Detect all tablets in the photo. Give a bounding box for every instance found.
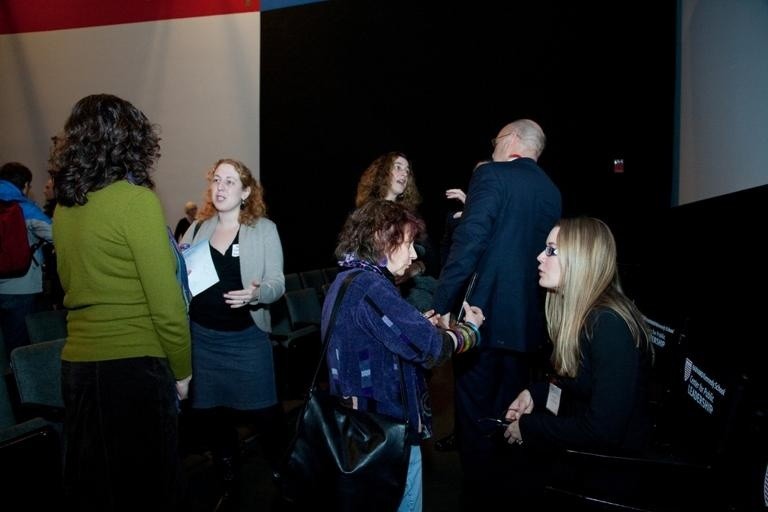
[445,272,479,326]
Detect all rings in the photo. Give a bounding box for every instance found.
[481,315,485,321]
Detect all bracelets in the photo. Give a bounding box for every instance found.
[448,321,481,354]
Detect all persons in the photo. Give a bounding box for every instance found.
[176,203,199,243]
[424,118,563,512]
[434,159,490,452]
[355,152,432,296]
[0,158,54,346]
[499,217,655,509]
[45,180,56,214]
[50,92,192,511]
[321,201,487,512]
[183,158,286,502]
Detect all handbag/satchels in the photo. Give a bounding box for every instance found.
[272,388,389,503]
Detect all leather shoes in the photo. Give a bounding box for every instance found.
[434,431,457,452]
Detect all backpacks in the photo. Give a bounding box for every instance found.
[0,199,33,280]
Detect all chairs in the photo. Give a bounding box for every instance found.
[543,309,736,512]
